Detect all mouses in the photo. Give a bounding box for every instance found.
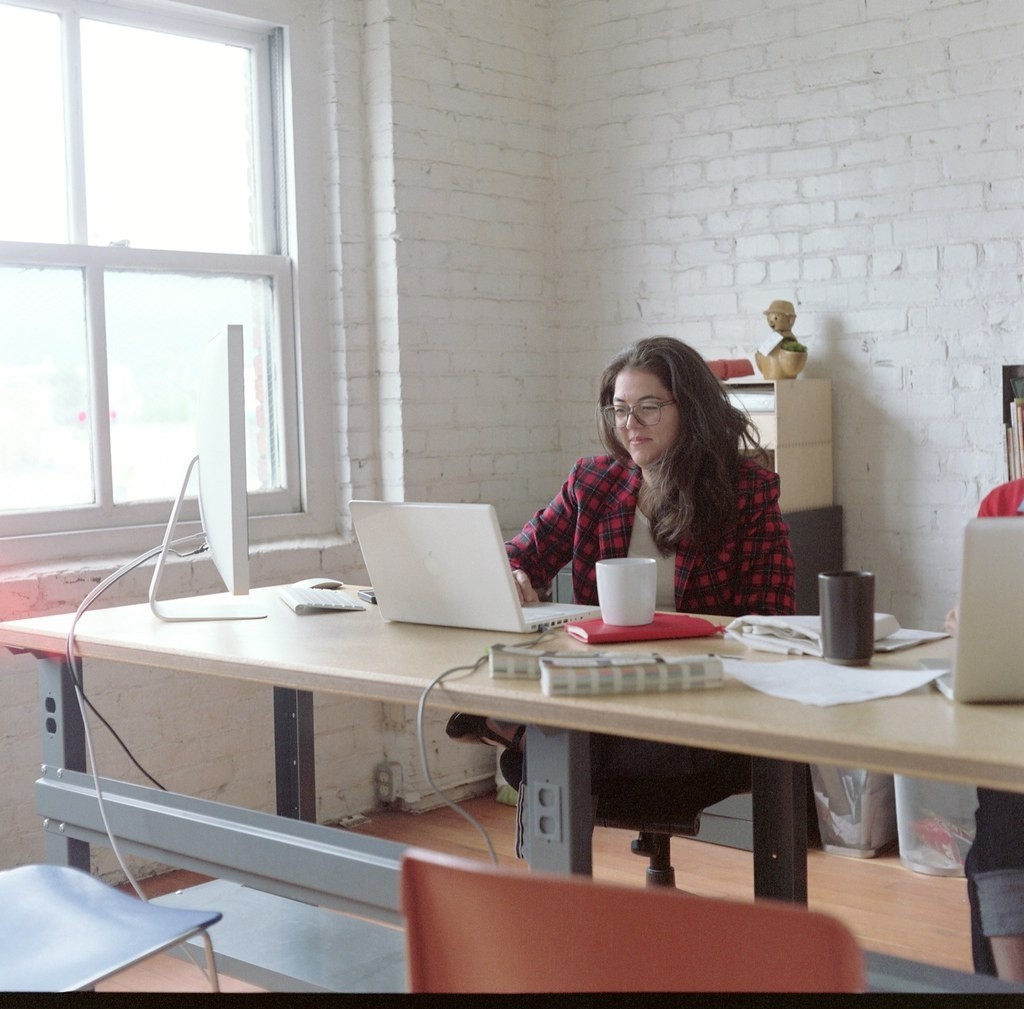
[295,578,344,590]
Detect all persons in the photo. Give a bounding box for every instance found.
[966,480,1024,984]
[446,336,796,795]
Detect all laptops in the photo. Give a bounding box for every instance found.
[349,500,602,634]
[922,516,1024,700]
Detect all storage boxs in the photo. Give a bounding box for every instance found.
[893,772,980,878]
[809,763,899,858]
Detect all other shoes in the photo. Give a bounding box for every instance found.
[445,711,527,753]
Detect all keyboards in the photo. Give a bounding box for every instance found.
[280,586,364,614]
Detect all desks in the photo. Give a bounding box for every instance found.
[0,583,1024,994]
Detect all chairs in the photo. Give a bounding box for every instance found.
[0,864,222,993]
[401,843,864,994]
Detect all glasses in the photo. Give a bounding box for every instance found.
[600,399,677,429]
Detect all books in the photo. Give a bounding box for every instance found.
[490,645,724,696]
[563,613,719,645]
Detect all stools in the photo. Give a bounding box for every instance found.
[496,748,752,891]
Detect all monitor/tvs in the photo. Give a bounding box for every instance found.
[148,324,270,622]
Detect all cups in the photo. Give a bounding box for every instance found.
[818,570,875,666]
[595,557,658,626]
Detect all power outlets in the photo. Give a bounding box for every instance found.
[376,762,402,802]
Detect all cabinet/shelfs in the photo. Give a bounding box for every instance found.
[674,379,833,849]
